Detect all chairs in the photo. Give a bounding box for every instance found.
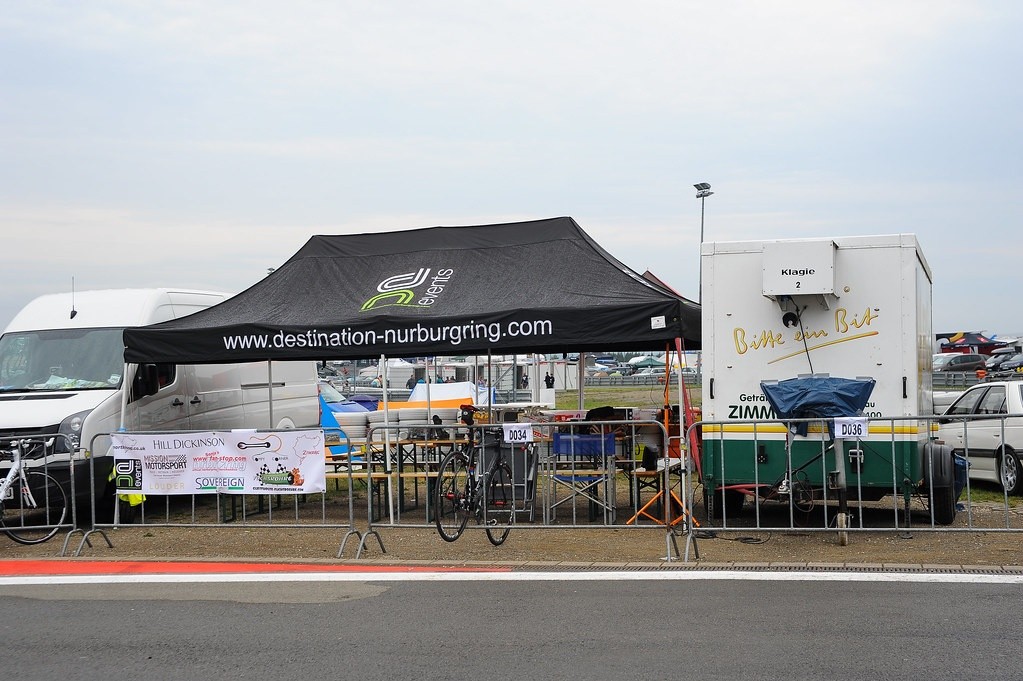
[547,432,617,524]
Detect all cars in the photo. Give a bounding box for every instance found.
[318,381,370,413]
[938,381,1023,493]
[985,354,1011,372]
[587,360,696,377]
[1000,353,1023,370]
[332,360,351,366]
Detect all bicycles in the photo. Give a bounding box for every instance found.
[1,437,67,545]
[434,404,516,546]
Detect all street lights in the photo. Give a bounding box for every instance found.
[693,182,714,305]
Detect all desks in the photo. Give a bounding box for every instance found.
[325,440,413,513]
[631,457,687,520]
[413,434,631,516]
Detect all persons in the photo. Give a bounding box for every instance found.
[461,376,467,382]
[478,376,484,386]
[343,367,348,374]
[544,372,555,388]
[521,373,528,389]
[437,374,456,383]
[370,375,382,388]
[424,376,431,383]
[405,375,424,389]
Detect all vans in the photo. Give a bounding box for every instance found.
[0,288,320,525]
[932,354,985,373]
[932,352,962,363]
[317,361,344,380]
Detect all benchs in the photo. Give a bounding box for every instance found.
[326,469,624,522]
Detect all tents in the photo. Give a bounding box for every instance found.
[114,217,701,524]
[940,334,1007,354]
[635,358,666,367]
